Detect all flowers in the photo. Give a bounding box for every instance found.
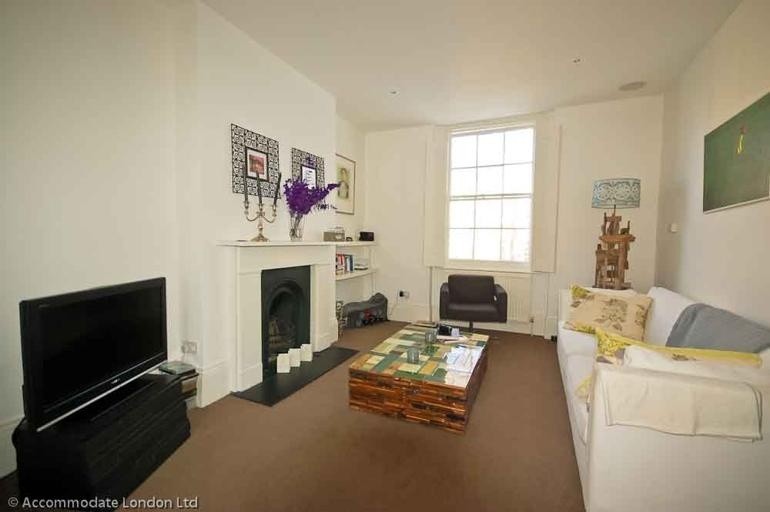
[283,176,340,236]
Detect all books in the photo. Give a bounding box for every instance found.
[336,253,354,275]
[353,265,368,270]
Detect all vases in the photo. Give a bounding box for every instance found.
[288,210,307,242]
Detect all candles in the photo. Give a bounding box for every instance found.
[256,170,262,204]
[241,164,248,202]
[273,172,281,205]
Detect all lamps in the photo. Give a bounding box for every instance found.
[592,178,640,290]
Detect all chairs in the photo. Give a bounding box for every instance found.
[439,274,508,328]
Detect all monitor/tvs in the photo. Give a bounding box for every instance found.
[19,276,169,434]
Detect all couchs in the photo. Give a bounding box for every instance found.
[556,285,770,512]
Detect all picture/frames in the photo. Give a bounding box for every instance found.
[335,153,356,215]
[300,164,317,190]
[244,146,269,182]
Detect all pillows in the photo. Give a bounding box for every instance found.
[623,345,770,386]
[574,326,763,398]
[562,281,652,343]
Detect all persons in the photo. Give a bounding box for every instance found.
[338,167,349,199]
[249,155,263,174]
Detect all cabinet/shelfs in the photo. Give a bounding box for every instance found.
[336,241,378,280]
[11,373,191,512]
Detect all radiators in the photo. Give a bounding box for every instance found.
[431,266,534,336]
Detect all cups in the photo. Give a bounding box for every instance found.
[408,348,419,363]
[425,330,437,345]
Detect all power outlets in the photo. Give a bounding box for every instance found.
[182,341,196,353]
